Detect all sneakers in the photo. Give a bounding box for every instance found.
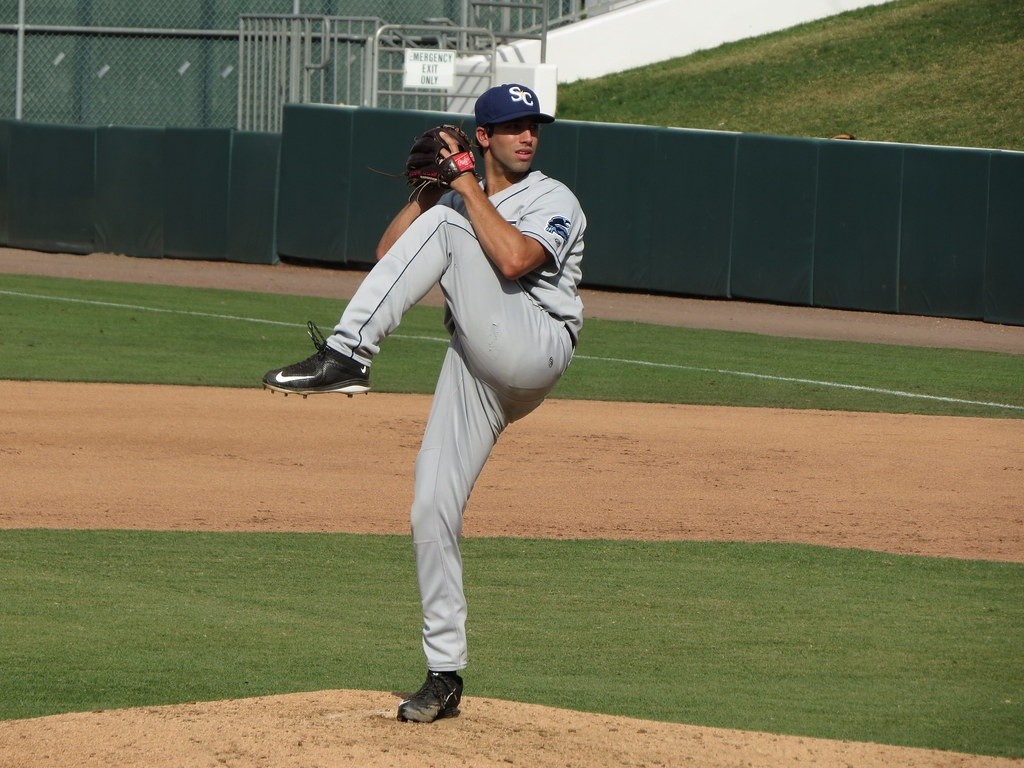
[260,320,372,400]
[397,671,462,723]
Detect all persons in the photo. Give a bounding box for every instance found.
[262,84,588,727]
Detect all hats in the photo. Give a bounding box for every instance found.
[474,83,554,128]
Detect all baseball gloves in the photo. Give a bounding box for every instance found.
[402,122,478,190]
